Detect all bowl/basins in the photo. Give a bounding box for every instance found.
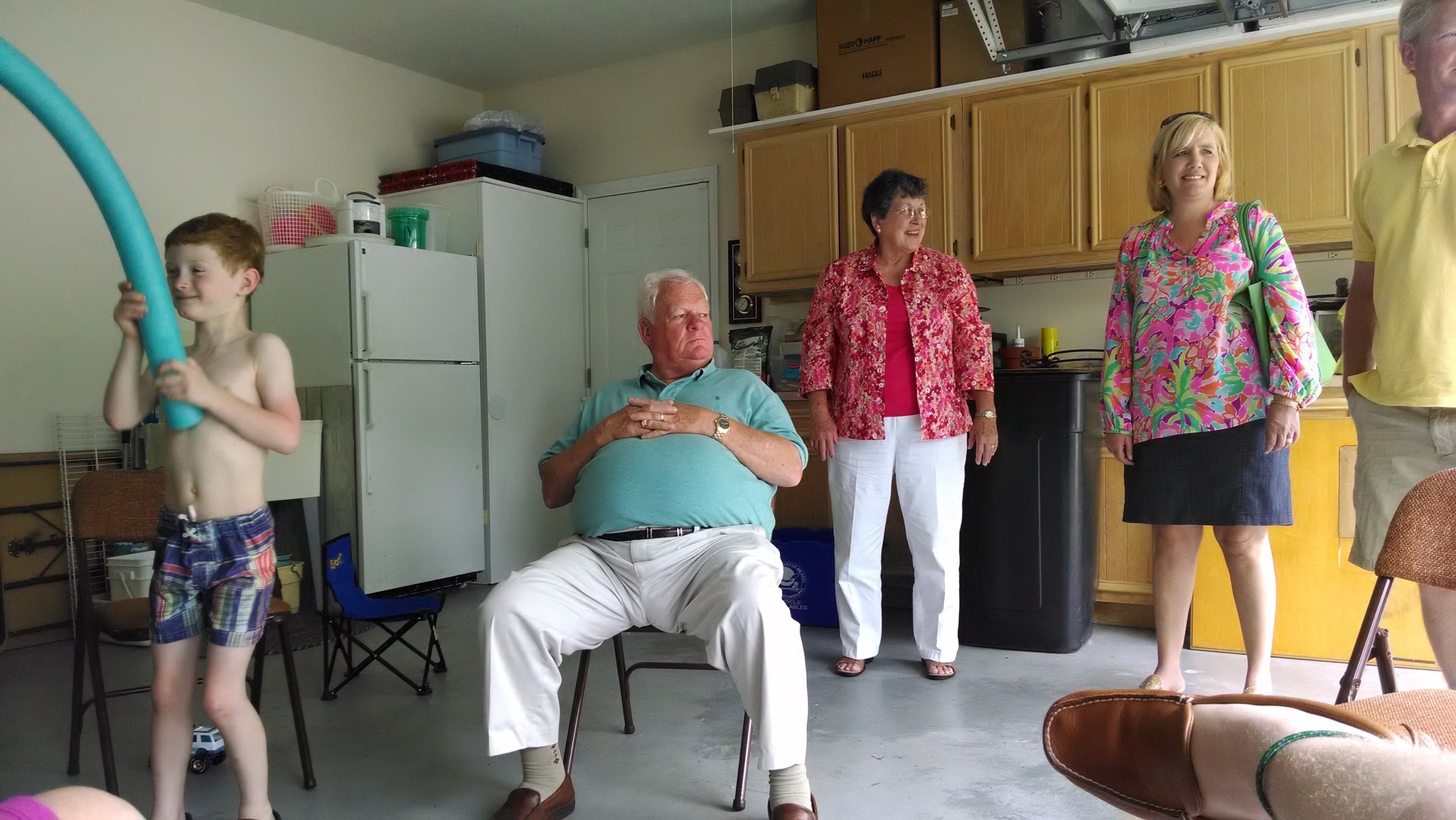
[998,347,1042,368]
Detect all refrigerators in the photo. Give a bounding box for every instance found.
[244,239,486,598]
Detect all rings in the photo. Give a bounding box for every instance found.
[991,445,995,448]
[1286,438,1293,444]
[660,413,663,421]
[642,420,647,428]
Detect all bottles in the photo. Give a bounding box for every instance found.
[713,341,733,369]
[1012,324,1024,349]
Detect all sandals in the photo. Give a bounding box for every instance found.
[835,657,874,677]
[921,657,956,679]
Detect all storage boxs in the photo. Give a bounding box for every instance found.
[754,59,818,120]
[718,84,759,127]
[940,0,1028,86]
[771,528,837,627]
[770,356,801,391]
[434,126,545,176]
[816,0,939,109]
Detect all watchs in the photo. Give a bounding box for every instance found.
[975,410,997,420]
[711,413,731,441]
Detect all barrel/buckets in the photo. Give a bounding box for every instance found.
[275,552,305,615]
[103,551,155,601]
[419,204,450,252]
[388,207,429,250]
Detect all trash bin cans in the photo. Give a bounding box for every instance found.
[959,369,1103,652]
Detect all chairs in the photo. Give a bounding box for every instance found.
[564,625,752,811]
[321,532,448,701]
[68,470,315,799]
[1336,468,1456,750]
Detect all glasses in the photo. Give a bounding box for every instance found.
[1161,112,1213,129]
[887,205,934,219]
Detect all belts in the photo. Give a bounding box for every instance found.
[599,526,705,542]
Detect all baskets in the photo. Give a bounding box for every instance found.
[258,178,341,253]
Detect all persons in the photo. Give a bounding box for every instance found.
[0,786,146,820]
[102,211,303,820]
[1096,110,1325,695]
[1043,690,1456,820]
[1339,0,1456,687]
[477,268,820,820]
[801,168,998,679]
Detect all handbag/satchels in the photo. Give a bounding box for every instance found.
[1235,203,1337,395]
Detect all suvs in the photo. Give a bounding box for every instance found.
[185,724,229,775]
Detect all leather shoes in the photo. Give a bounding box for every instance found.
[489,772,575,820]
[1042,690,1437,820]
[765,792,821,820]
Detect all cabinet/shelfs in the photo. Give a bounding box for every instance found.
[740,20,1421,297]
[379,176,585,584]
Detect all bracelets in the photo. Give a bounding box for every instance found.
[1270,400,1301,413]
